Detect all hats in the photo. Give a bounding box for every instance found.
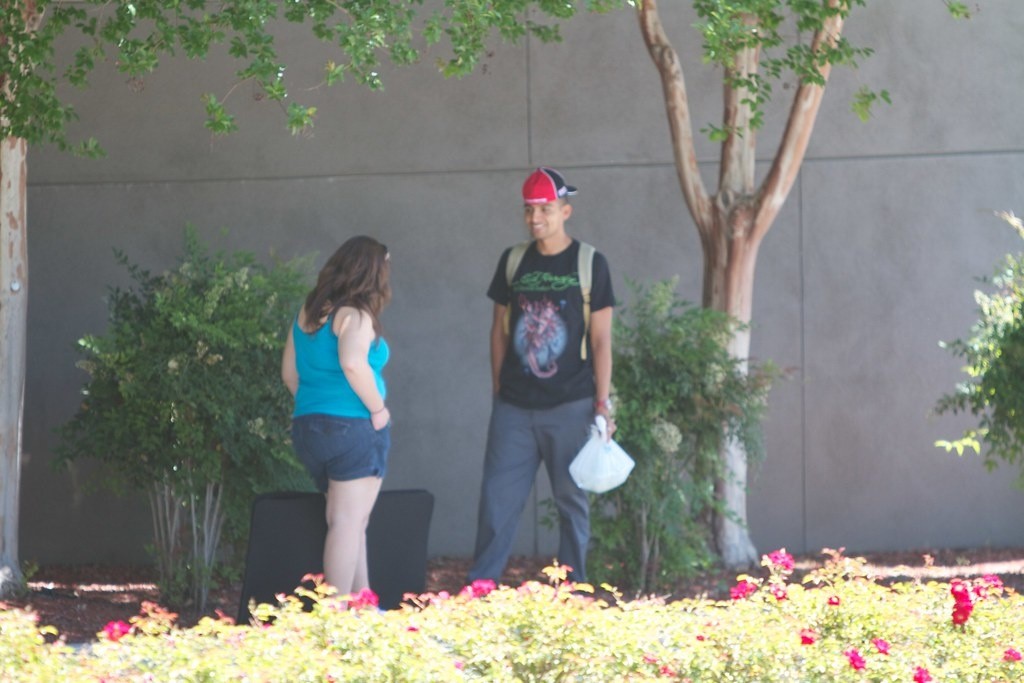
[522,166,578,205]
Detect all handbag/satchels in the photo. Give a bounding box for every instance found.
[569,401,635,494]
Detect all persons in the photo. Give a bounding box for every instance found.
[466,168,617,593]
[282,235,392,609]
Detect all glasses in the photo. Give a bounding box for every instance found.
[381,245,390,261]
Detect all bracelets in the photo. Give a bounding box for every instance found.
[370,406,386,415]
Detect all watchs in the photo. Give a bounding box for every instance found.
[593,399,612,411]
